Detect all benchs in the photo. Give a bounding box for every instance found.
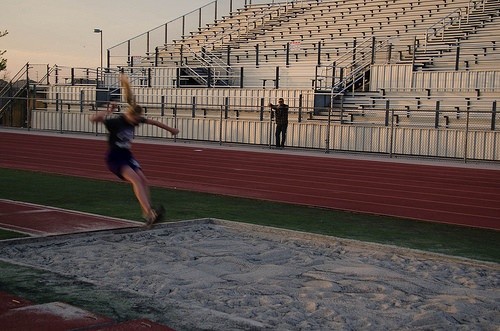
[36,0,500,131]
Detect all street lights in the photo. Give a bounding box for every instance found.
[93,28,103,87]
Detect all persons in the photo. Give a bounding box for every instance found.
[89,75,180,227]
[268,98,289,149]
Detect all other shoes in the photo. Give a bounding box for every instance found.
[146,207,166,227]
